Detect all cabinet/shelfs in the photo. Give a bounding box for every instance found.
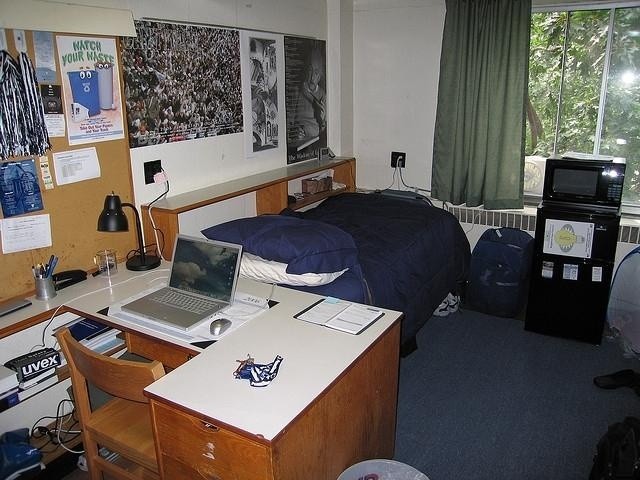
[141,157,356,262]
[524,202,623,344]
[0,262,404,480]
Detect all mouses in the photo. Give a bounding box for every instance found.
[210,318,232,336]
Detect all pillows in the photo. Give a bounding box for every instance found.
[199,213,356,289]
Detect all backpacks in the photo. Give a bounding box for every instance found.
[464,227,535,317]
[589,417,640,480]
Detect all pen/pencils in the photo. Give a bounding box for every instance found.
[32,254,58,278]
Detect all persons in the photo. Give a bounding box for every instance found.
[293,48,327,138]
[119,19,244,148]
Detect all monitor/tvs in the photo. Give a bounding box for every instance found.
[542,154,626,213]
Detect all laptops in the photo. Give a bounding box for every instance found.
[121,234,243,332]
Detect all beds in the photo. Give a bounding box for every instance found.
[251,191,467,322]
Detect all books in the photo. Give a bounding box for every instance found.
[68,317,128,359]
[18,367,59,402]
[292,296,385,335]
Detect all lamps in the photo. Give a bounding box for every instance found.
[96,193,160,272]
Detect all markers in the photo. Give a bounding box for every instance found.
[92,264,114,277]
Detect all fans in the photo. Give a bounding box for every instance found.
[524,156,546,195]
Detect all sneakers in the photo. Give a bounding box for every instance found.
[432,300,449,316]
[447,295,459,312]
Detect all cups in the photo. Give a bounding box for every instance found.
[95,248,117,276]
[35,276,57,301]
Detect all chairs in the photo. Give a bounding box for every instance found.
[55,329,166,480]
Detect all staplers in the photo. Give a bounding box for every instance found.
[53,270,87,291]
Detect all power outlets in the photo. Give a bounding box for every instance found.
[391,152,406,168]
[144,159,162,184]
